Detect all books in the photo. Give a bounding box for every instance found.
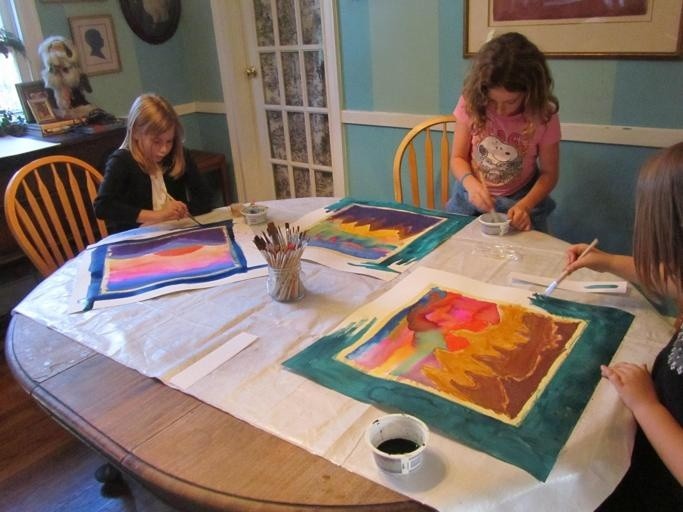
[70,118,127,134]
[23,118,82,138]
[52,103,98,120]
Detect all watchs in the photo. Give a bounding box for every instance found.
[457,173,474,192]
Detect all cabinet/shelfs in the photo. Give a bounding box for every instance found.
[0,131,129,269]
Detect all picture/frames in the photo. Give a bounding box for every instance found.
[15,81,44,123]
[26,88,55,109]
[120,0,182,46]
[27,99,56,124]
[463,0,683,62]
[68,14,122,77]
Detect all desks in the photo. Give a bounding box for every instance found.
[4,197,653,510]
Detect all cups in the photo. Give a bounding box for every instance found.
[267,263,305,302]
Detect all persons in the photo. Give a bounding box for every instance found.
[443,31,562,235]
[85,28,105,60]
[143,0,170,37]
[92,93,188,236]
[565,142,683,489]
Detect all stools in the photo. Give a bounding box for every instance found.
[189,148,232,207]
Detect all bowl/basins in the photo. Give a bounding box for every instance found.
[365,412,430,475]
[240,205,270,225]
[479,213,513,235]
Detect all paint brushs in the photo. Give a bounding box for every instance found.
[545,238,598,299]
[160,188,203,226]
[478,170,500,222]
[250,222,310,301]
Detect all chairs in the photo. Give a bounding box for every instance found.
[3,154,106,277]
[393,114,459,210]
